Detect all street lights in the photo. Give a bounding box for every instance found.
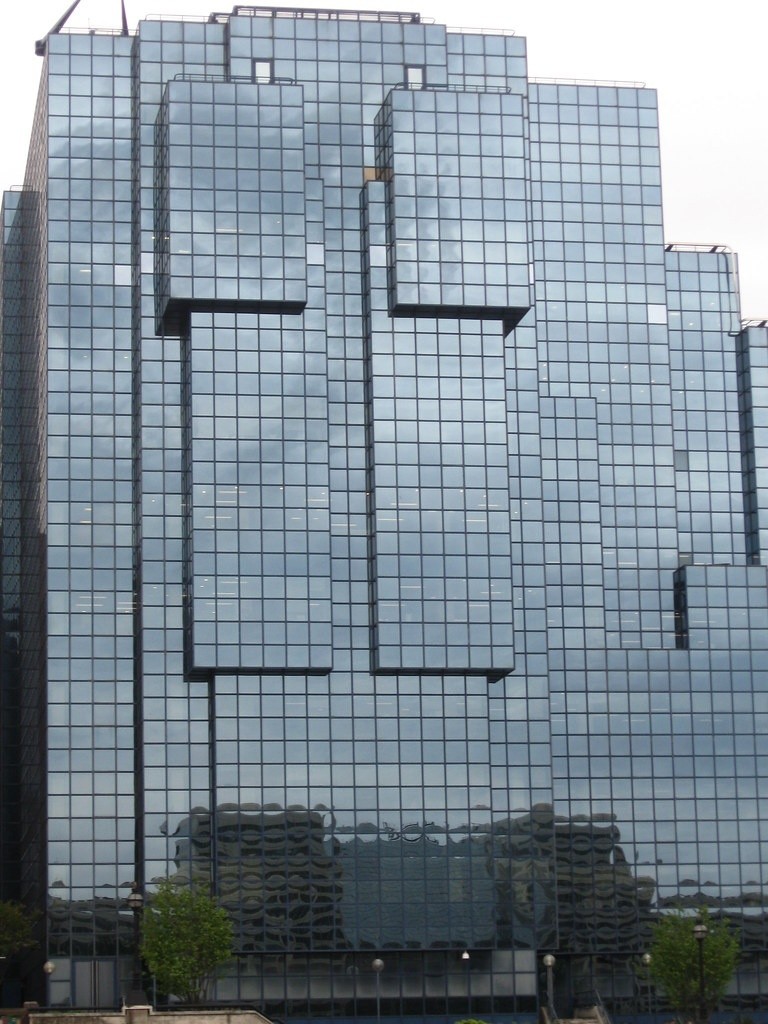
[691,916,710,1024]
[125,887,147,1006]
